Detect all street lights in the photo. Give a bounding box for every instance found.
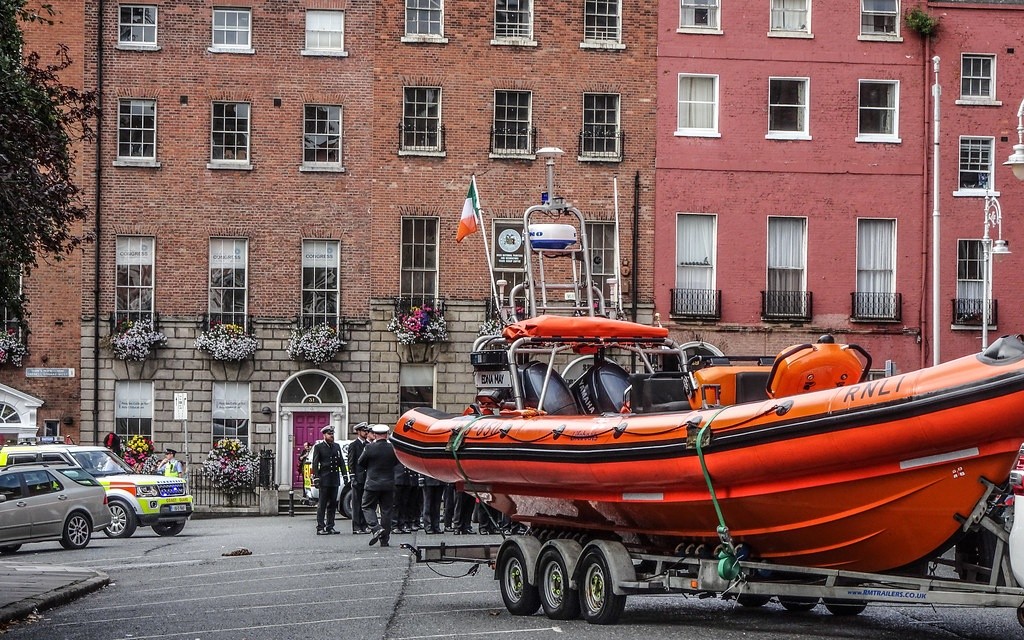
[979,166,1008,352]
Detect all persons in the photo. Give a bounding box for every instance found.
[298,441,310,498]
[348,421,528,535]
[312,426,348,535]
[358,424,395,547]
[157,449,182,478]
[104,432,120,457]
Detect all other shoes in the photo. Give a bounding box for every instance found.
[380,541,389,547]
[369,528,385,546]
[353,526,371,534]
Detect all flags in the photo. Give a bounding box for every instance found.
[456,180,479,243]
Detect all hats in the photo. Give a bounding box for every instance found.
[320,426,335,434]
[372,424,389,433]
[164,448,176,454]
[366,424,375,431]
[353,422,368,431]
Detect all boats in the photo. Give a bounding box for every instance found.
[389,144,1024,572]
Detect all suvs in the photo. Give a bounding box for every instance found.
[298,438,444,521]
[0,436,196,556]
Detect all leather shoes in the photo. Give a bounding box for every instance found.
[434,527,444,534]
[411,523,419,531]
[400,525,411,534]
[454,524,525,536]
[444,526,454,531]
[317,529,328,535]
[425,528,433,534]
[326,528,340,534]
[392,528,404,534]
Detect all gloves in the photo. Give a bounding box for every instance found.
[313,479,320,488]
[352,478,358,487]
[343,476,348,485]
